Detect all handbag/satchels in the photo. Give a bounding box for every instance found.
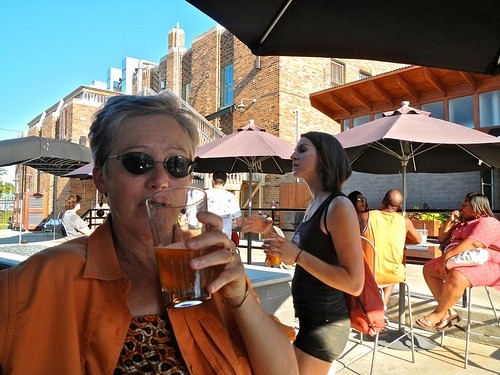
[443,242,489,270]
[325,194,385,336]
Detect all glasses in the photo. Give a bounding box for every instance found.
[107,150,198,178]
[356,197,367,202]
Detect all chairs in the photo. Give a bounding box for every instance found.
[338,235,414,375]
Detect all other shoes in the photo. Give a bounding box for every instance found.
[383,310,390,326]
[366,333,377,341]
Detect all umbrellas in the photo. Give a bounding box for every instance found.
[185,0,500,77]
[332,102,500,331]
[61,163,99,209]
[191,119,298,266]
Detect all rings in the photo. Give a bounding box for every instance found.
[223,246,239,265]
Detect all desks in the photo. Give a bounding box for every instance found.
[376,245,441,352]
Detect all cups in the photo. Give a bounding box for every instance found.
[262,238,283,265]
[420,230,428,244]
[145,188,212,308]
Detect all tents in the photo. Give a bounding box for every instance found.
[0,135,96,246]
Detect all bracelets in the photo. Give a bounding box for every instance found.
[295,249,303,263]
[220,276,250,310]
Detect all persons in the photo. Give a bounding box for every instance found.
[204,170,244,241]
[415,192,500,332]
[348,190,369,213]
[235,131,365,375]
[62,194,89,235]
[0,95,299,375]
[358,189,421,341]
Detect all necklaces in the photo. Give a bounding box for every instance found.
[303,192,326,222]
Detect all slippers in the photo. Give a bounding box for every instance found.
[416,315,435,330]
[435,309,460,332]
